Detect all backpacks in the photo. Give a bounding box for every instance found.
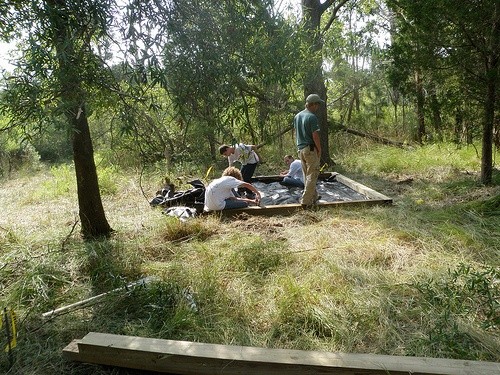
[162,206,197,223]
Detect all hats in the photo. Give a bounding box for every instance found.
[306,93,325,103]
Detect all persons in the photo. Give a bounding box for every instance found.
[292,94,325,211]
[218,143,261,199]
[204,166,261,211]
[279,154,305,187]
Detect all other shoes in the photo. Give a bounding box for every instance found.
[318,196,321,200]
[302,204,312,209]
[237,191,245,196]
[246,194,253,199]
